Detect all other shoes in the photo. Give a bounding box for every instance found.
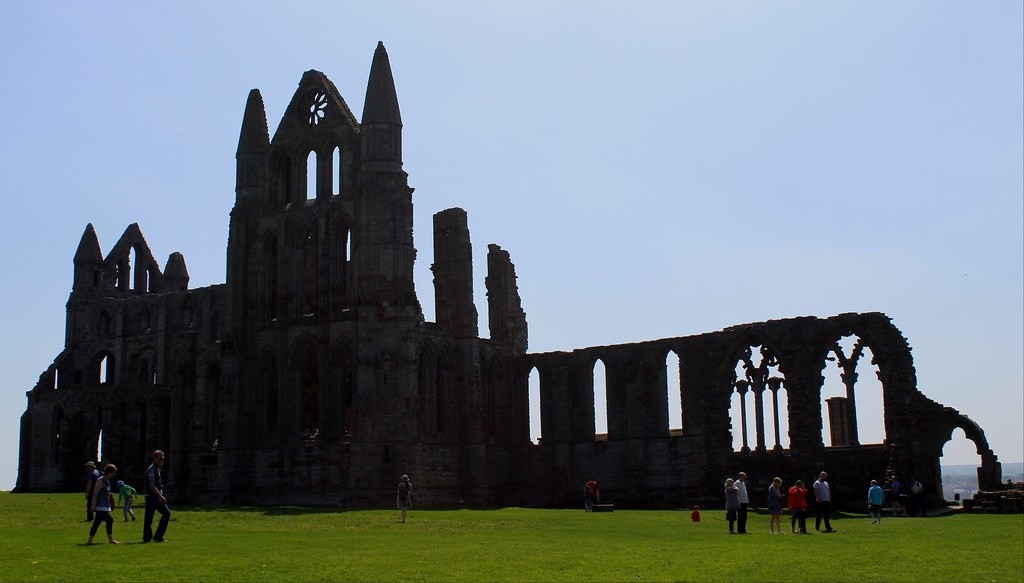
[826,528,836,532]
[730,531,735,534]
[872,520,877,524]
[792,528,798,533]
[778,529,786,535]
[771,530,777,534]
[132,516,135,521]
[153,536,167,542]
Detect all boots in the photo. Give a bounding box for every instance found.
[87,536,99,546]
[107,533,120,544]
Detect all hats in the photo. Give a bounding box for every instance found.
[401,475,409,482]
[117,481,124,487]
[86,461,96,468]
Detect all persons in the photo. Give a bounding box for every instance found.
[397,474,412,522]
[585,481,599,512]
[867,480,884,524]
[725,478,739,534]
[117,481,137,522]
[890,475,901,507]
[89,464,120,544]
[788,480,807,534]
[691,505,700,522]
[813,471,834,531]
[768,477,786,535]
[143,450,170,543]
[86,461,99,521]
[909,477,926,516]
[734,472,749,533]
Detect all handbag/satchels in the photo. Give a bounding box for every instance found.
[726,512,737,521]
